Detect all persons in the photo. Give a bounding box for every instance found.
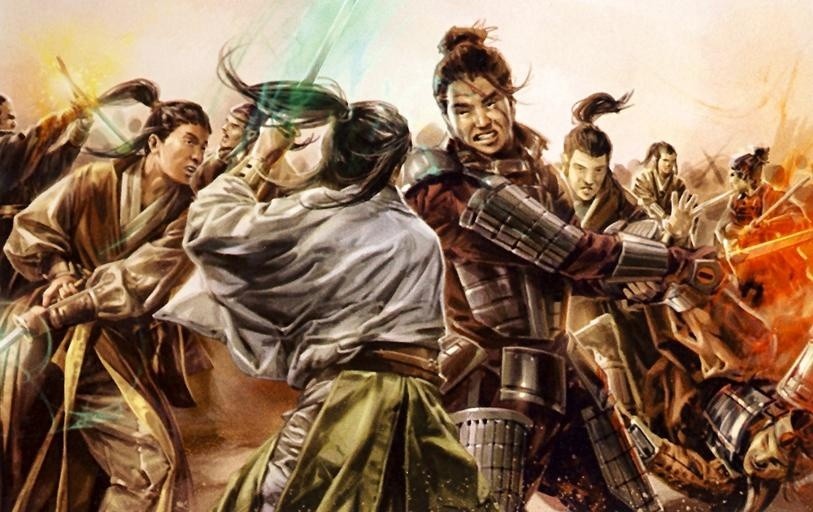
[0,18,813,512]
